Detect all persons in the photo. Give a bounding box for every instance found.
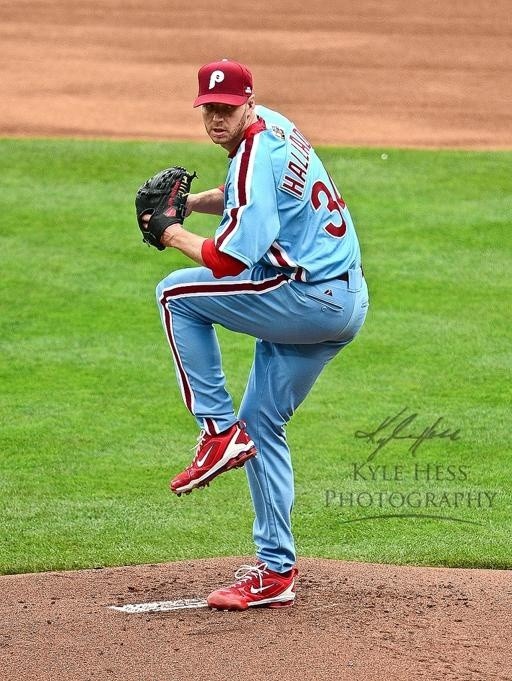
[140,59,369,611]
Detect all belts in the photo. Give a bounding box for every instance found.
[336,266,364,281]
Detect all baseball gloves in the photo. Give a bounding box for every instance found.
[135,165,198,250]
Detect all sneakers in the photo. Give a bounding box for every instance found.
[207,560,298,611]
[193,59,255,108]
[169,421,257,497]
